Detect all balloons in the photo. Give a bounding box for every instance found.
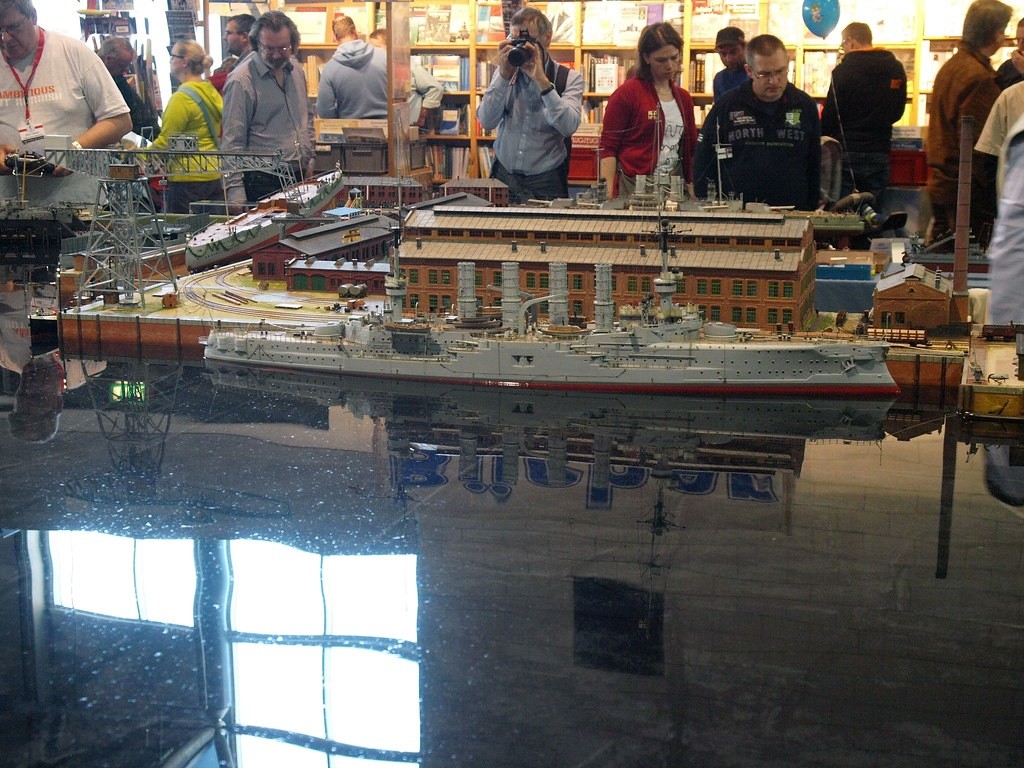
[802,0,840,39]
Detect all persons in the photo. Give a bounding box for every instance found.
[0,0,134,177]
[695,33,820,211]
[222,9,316,215]
[0,280,109,442]
[369,28,444,135]
[223,13,257,68]
[206,57,239,143]
[315,15,387,120]
[477,7,583,201]
[94,38,160,142]
[926,0,1024,352]
[121,38,227,215]
[713,27,749,104]
[820,21,907,212]
[598,21,697,200]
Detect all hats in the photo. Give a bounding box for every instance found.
[715,26,745,45]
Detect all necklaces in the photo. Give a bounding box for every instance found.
[657,88,672,95]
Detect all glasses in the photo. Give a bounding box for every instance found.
[747,64,789,80]
[256,38,294,54]
[169,51,187,60]
[0,17,26,36]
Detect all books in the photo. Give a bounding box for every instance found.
[584,0,1024,129]
[375,2,634,180]
[165,0,200,55]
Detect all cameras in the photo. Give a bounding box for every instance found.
[509,27,536,67]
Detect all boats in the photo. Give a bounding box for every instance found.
[507,103,865,246]
[198,219,901,397]
[185,163,347,274]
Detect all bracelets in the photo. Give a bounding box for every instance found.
[72,140,83,150]
[541,82,555,96]
